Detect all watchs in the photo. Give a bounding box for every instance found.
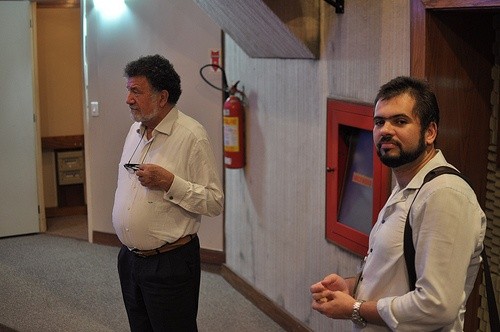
[351,300,367,329]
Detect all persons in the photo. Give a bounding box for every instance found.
[310,76,487,332]
[111,54,224,332]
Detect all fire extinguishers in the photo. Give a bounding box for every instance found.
[222,79,246,170]
